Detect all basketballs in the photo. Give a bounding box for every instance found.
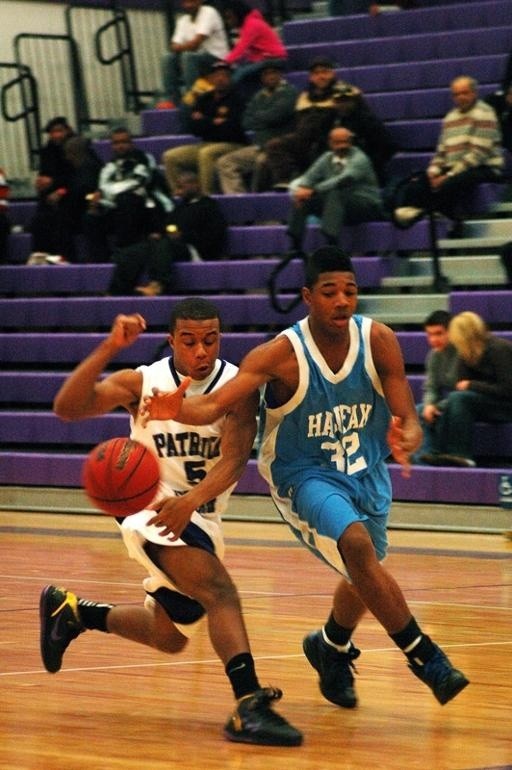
[80,436,160,518]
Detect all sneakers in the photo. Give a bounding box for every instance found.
[304,626,360,708]
[419,452,476,467]
[28,251,69,265]
[40,585,81,672]
[408,643,468,705]
[134,280,162,296]
[222,687,303,745]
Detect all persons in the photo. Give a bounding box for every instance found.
[420,312,512,470]
[26,0,397,294]
[423,75,507,239]
[140,243,472,708]
[413,309,467,465]
[39,296,306,746]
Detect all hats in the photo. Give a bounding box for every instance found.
[204,53,337,75]
